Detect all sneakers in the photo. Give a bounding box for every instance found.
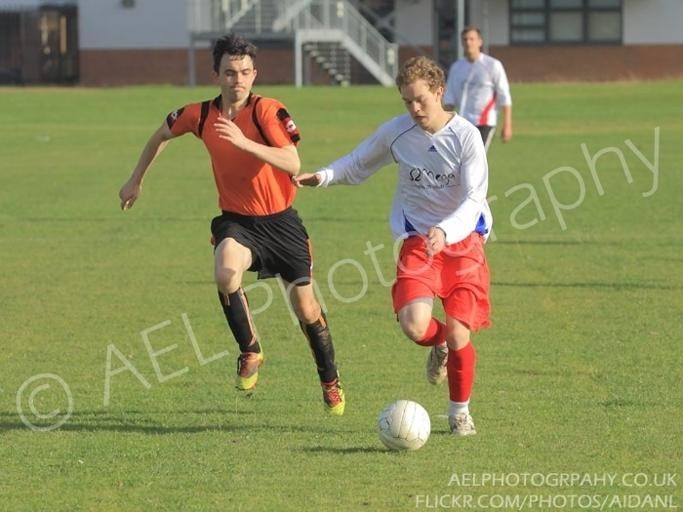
[427,345,449,384]
[449,411,476,436]
[321,369,345,415]
[235,342,263,390]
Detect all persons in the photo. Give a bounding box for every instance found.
[119,34,345,418]
[292,55,493,437]
[441,25,513,152]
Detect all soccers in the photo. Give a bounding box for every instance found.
[378,400,431,450]
